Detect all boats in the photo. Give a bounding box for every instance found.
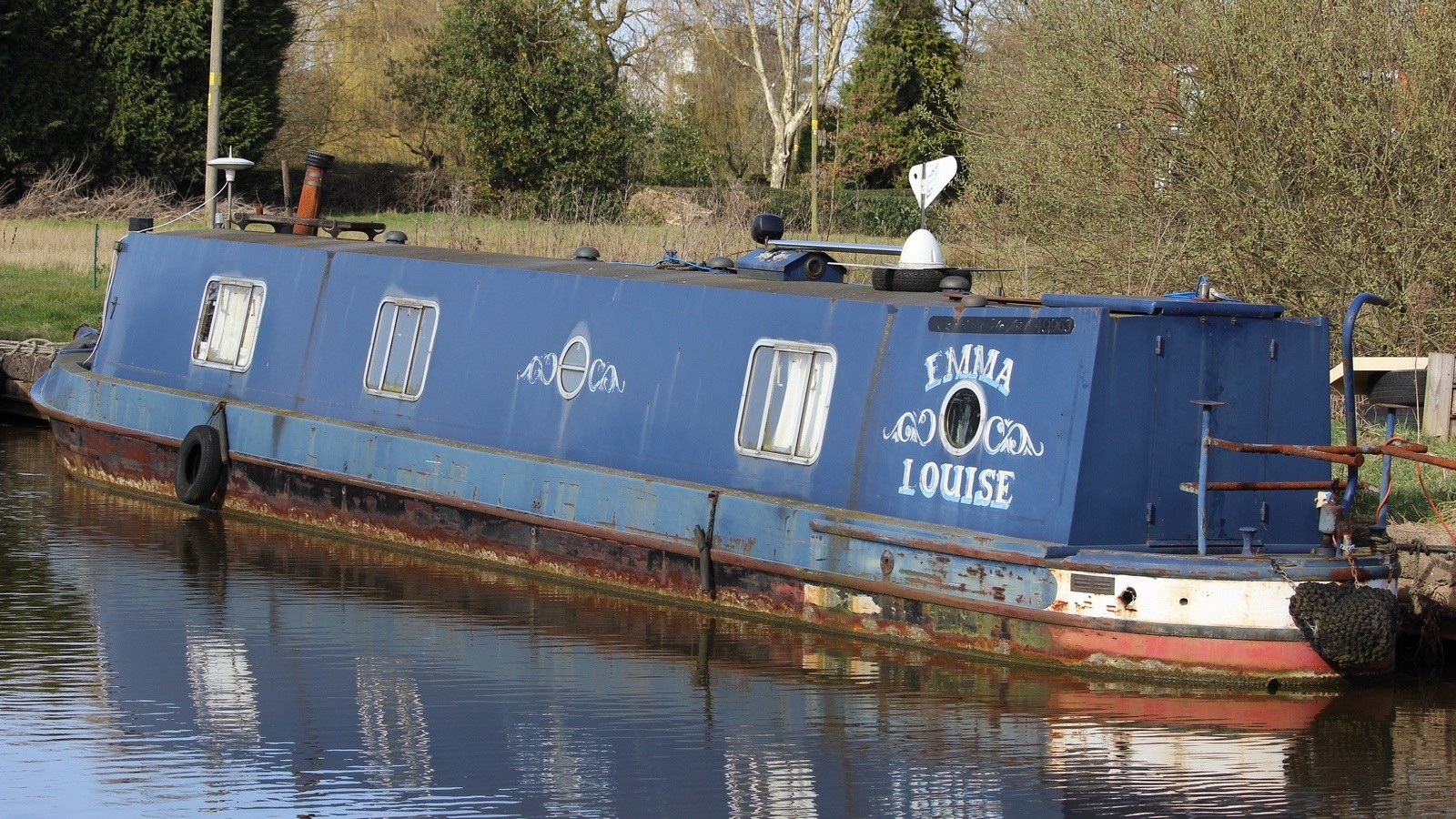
[28,140,1455,694]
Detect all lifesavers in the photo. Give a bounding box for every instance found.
[172,425,224,504]
[173,517,221,575]
